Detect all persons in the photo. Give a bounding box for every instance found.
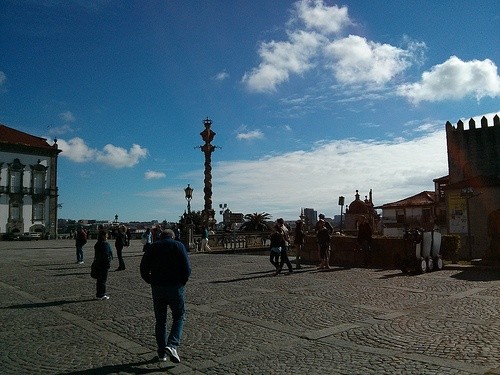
[115,224,211,363]
[90,229,113,300]
[269,213,334,276]
[358,214,372,262]
[76,213,87,265]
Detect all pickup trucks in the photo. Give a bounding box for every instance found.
[24,231,41,241]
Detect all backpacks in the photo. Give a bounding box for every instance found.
[122,233,130,247]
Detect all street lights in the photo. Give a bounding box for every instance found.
[194,117,222,217]
[338,195,345,231]
[184,184,194,213]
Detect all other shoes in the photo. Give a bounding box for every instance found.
[164,346,181,363]
[98,296,110,300]
[115,268,122,271]
[277,267,281,273]
[317,266,329,269]
[76,260,83,264]
[158,356,167,362]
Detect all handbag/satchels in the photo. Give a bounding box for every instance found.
[90,256,98,278]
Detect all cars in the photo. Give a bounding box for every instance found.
[9,233,26,241]
